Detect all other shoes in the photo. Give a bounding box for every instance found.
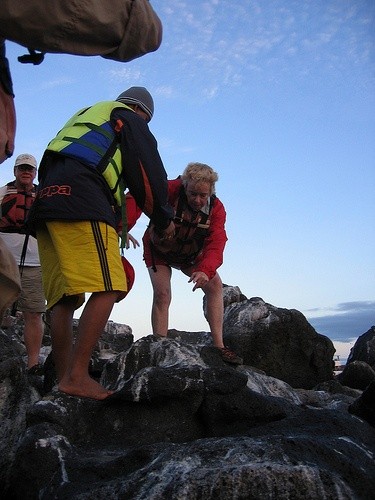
[30,364,44,377]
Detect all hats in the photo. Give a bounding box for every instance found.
[14,153,37,169]
[116,86,154,123]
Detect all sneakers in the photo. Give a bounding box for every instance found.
[211,346,243,364]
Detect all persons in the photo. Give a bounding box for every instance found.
[0,0,163,161]
[24,87,176,398]
[141,161,245,366]
[0,154,48,374]
